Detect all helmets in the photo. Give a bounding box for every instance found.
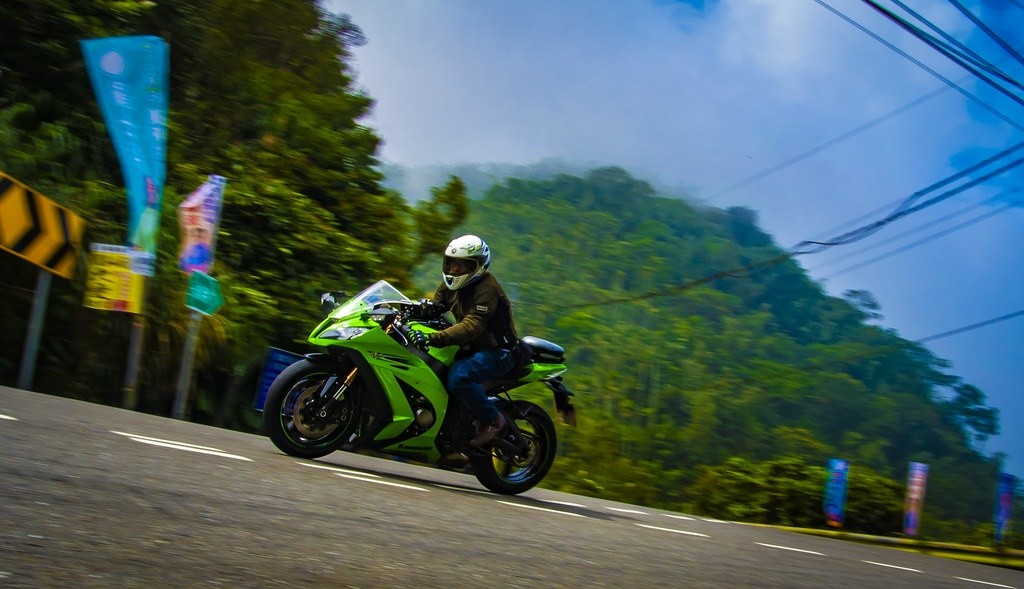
[441,235,490,291]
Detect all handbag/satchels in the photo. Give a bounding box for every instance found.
[512,340,533,370]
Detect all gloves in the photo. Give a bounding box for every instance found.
[407,330,431,347]
[380,301,393,310]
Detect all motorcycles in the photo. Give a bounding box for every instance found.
[263,280,577,495]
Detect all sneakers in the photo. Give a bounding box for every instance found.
[470,412,506,447]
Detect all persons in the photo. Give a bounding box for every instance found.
[405,234,522,446]
